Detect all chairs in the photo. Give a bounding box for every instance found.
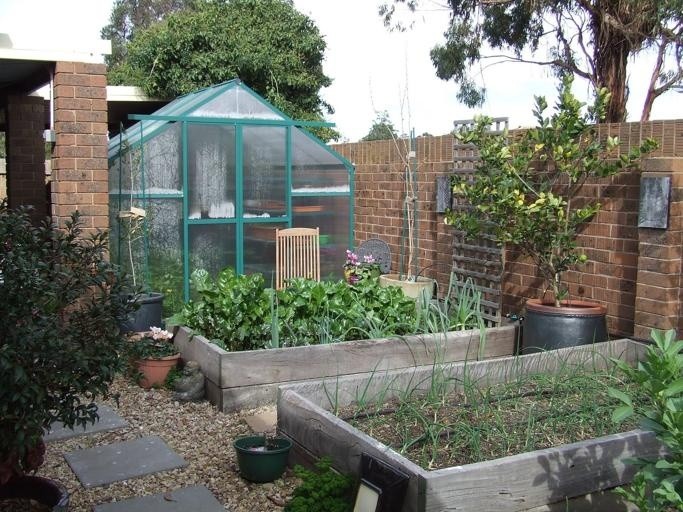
[275,225,319,290]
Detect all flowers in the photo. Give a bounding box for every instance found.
[342,249,374,282]
[132,325,181,360]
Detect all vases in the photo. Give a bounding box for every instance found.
[232,435,292,484]
[136,354,180,389]
[344,270,354,282]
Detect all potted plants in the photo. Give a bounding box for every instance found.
[367,43,434,334]
[110,141,164,336]
[1,196,149,510]
[442,82,660,355]
